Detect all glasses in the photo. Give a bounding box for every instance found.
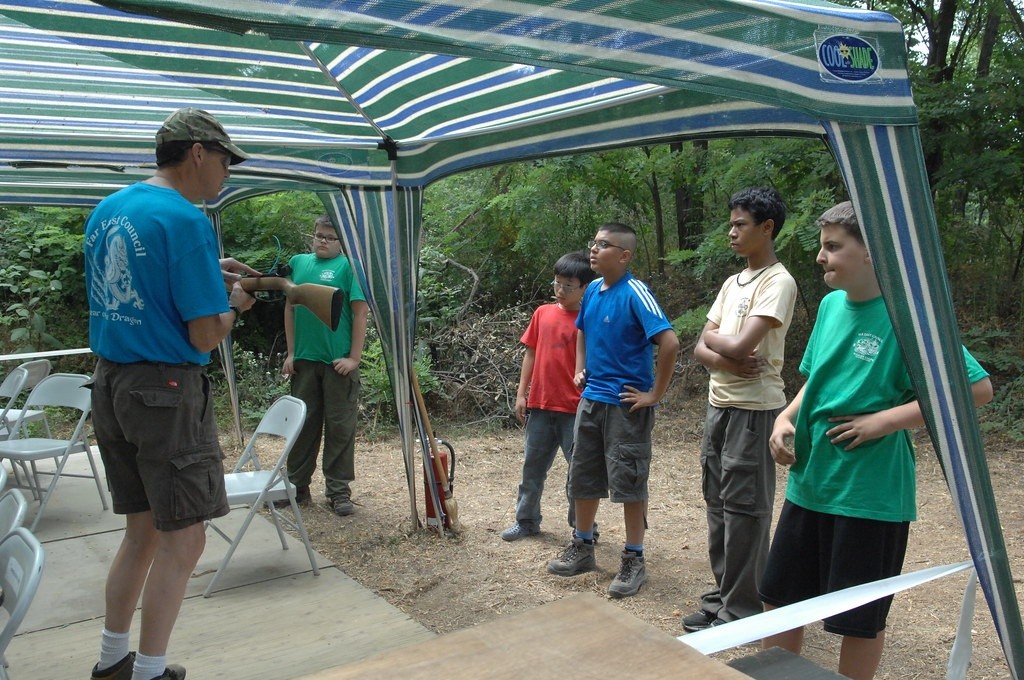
[314,233,340,243]
[588,239,626,252]
[210,146,231,169]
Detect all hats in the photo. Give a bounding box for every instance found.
[156,107,251,166]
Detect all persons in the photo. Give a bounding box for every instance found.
[500,251,599,544]
[83,108,264,679]
[545,222,680,601]
[681,188,798,644]
[758,199,992,680]
[261,216,370,516]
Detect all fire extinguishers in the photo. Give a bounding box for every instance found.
[423,430,455,534]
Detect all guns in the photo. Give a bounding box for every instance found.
[224,263,345,333]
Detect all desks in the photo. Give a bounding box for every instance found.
[290,588,755,680]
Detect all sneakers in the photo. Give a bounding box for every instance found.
[263,485,308,508]
[501,515,543,541]
[706,619,726,630]
[330,492,366,517]
[607,551,648,596]
[682,608,716,631]
[546,538,596,576]
[151,664,186,680]
[90,651,136,680]
[567,507,599,541]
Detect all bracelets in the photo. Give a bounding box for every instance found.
[229,306,242,323]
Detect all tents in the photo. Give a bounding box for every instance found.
[0,0,1024,680]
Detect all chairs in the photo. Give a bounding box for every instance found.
[203,396,320,599]
[0,359,111,680]
